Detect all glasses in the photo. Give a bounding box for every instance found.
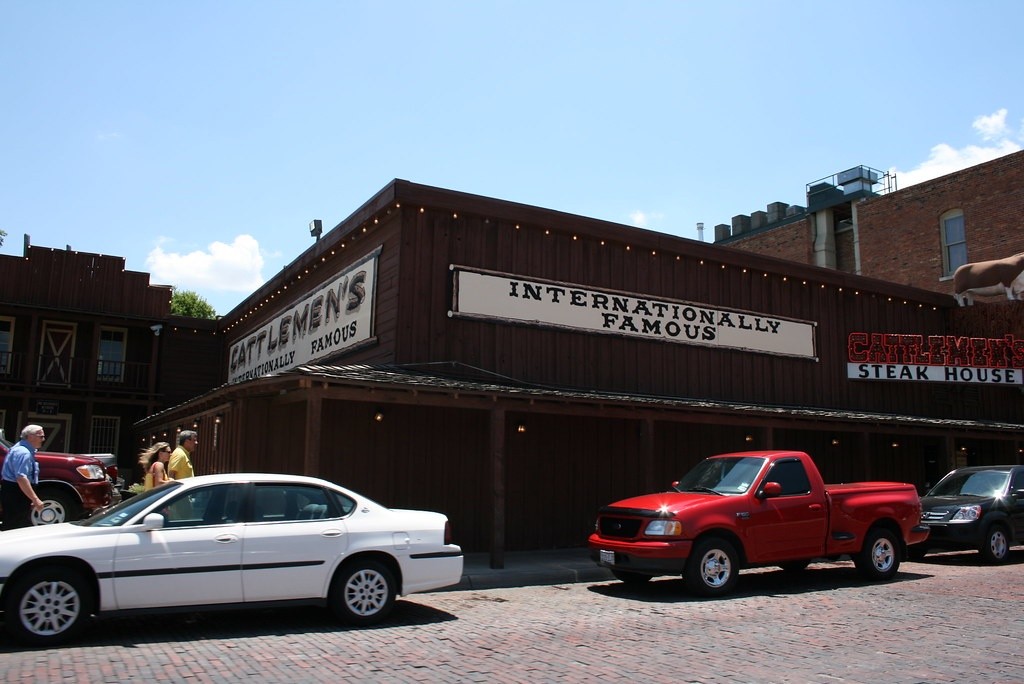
[162,451,172,454]
[36,436,45,439]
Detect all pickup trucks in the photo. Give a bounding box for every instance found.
[588,451,930,599]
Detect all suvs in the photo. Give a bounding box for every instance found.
[0,428,114,525]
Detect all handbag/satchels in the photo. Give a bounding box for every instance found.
[144,462,167,492]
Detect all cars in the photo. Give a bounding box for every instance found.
[0,473,464,647]
[921,465,1024,564]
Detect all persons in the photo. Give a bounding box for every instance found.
[166,430,199,521]
[138,441,175,490]
[0,425,46,532]
